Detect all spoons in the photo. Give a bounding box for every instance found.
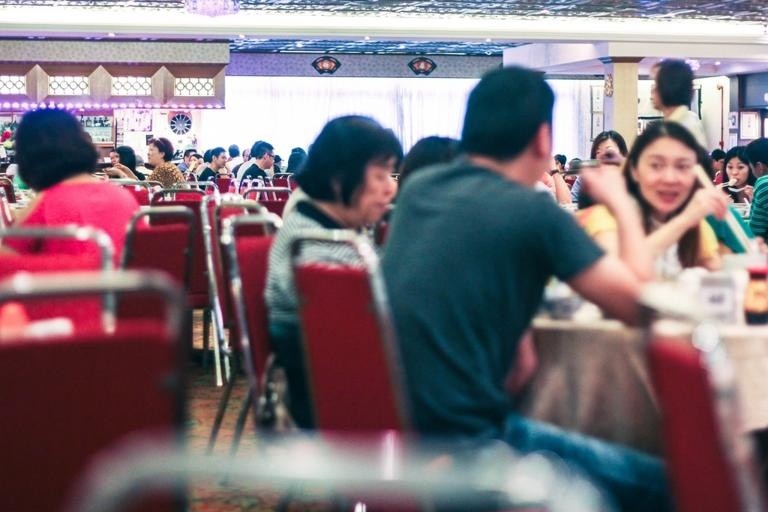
[728,187,746,193]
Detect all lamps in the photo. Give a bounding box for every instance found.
[406,57,437,76]
[312,55,341,75]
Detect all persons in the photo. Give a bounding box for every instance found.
[0,108,273,272]
[263,55,768,511]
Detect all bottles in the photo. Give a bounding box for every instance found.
[80,115,110,126]
[743,268,767,325]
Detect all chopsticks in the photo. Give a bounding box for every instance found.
[714,179,730,189]
